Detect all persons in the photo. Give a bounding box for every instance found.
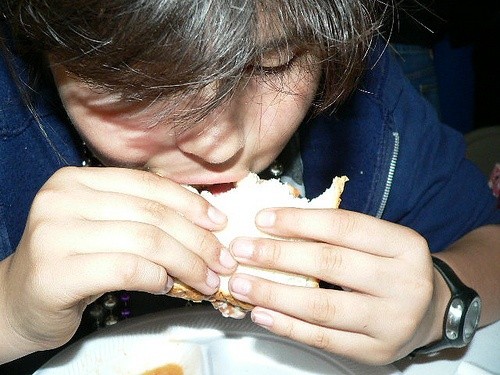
[1,3,500,365]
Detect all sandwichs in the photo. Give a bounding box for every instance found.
[143,172,347,317]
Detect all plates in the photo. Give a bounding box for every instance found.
[32,306,401,375]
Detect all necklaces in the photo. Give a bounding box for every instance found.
[442,287,481,349]
[76,143,293,329]
